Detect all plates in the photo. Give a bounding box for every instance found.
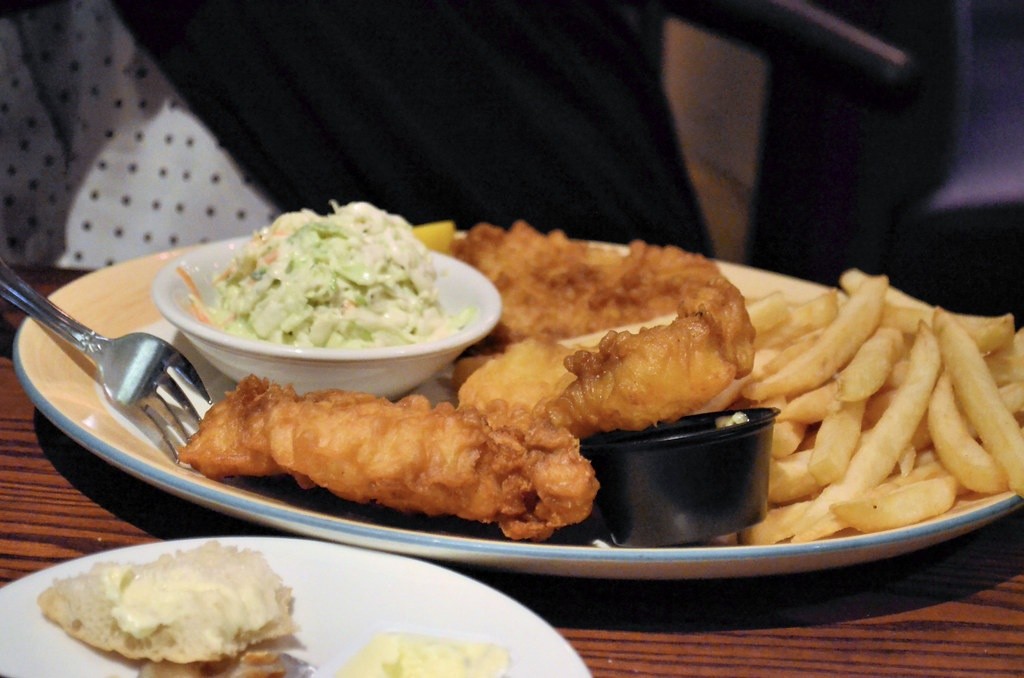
[0,535,593,678]
[12,238,1024,581]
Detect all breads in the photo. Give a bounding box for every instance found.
[36,539,300,678]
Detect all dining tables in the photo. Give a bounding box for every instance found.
[0,262,1023,678]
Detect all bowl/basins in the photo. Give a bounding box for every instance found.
[581,407,779,547]
[152,234,503,402]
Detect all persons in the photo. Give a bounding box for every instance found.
[0,0,279,274]
[643,1,903,290]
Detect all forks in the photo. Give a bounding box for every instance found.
[0,259,211,467]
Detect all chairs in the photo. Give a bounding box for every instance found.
[614,1,917,277]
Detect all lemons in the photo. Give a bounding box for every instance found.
[413,220,456,253]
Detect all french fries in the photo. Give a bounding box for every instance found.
[450,268,1024,546]
